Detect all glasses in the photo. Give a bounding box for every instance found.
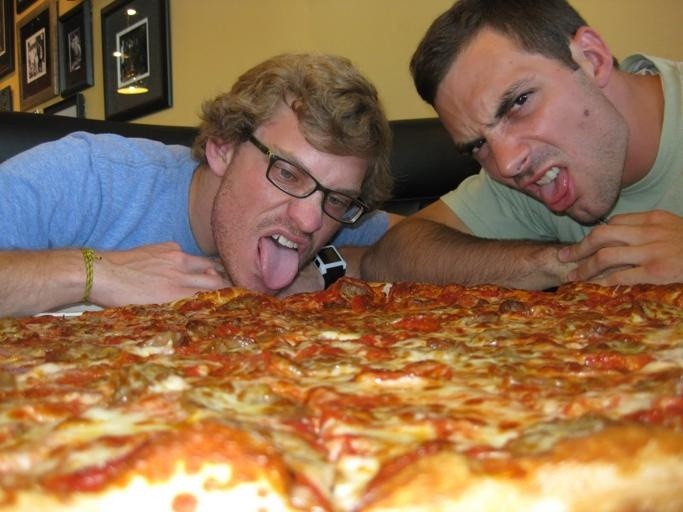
[247,133,370,226]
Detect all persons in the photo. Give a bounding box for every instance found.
[68,31,80,64]
[360,0,682,291]
[0,50,412,317]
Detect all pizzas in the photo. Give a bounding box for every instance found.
[0,276,683,512]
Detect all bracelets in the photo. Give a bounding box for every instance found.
[76,245,99,307]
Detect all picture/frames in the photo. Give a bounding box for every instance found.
[0,0,172,123]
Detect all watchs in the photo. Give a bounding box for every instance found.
[309,244,348,290]
